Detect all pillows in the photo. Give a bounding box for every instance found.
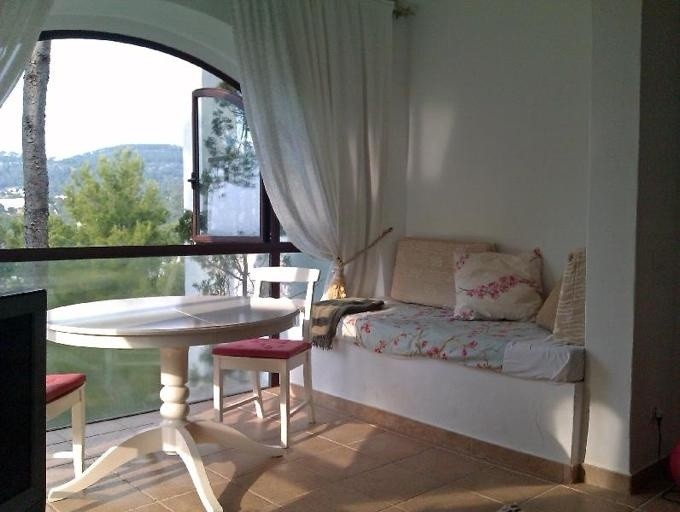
[452,246,547,321]
[390,240,497,310]
[535,249,586,343]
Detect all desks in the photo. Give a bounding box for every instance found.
[47,294,300,511]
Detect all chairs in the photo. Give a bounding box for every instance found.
[211,267,321,449]
[46,373,86,478]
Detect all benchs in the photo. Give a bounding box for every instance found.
[279,296,585,468]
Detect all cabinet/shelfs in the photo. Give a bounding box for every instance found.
[0,288,46,511]
